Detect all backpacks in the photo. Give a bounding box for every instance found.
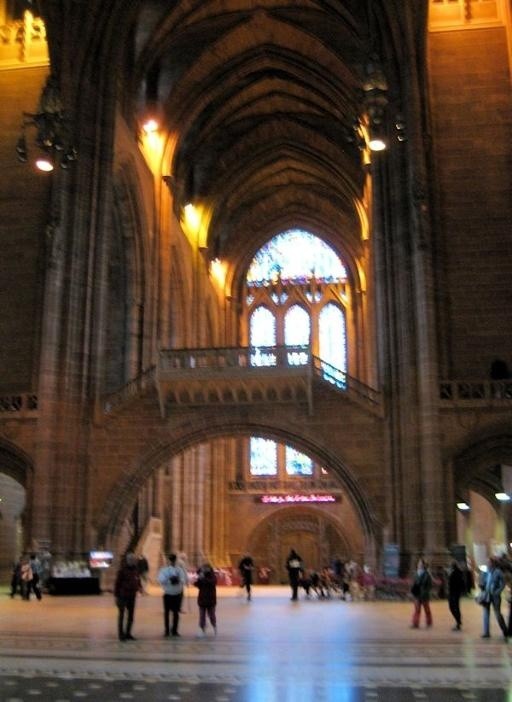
[21,565,33,582]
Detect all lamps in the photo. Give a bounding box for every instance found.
[327,84,411,160]
[12,104,81,174]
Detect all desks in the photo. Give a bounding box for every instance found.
[45,574,104,598]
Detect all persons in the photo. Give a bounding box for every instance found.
[475,556,510,640]
[408,555,434,630]
[193,562,218,640]
[115,552,190,643]
[283,545,377,603]
[9,553,43,601]
[237,549,256,602]
[446,559,464,631]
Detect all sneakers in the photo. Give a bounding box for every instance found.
[409,624,419,630]
[451,622,462,631]
[125,632,135,640]
[172,631,180,637]
[480,634,490,639]
[118,633,126,642]
[164,632,170,637]
[425,623,433,630]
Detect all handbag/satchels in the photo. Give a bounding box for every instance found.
[474,591,490,605]
[410,583,421,598]
